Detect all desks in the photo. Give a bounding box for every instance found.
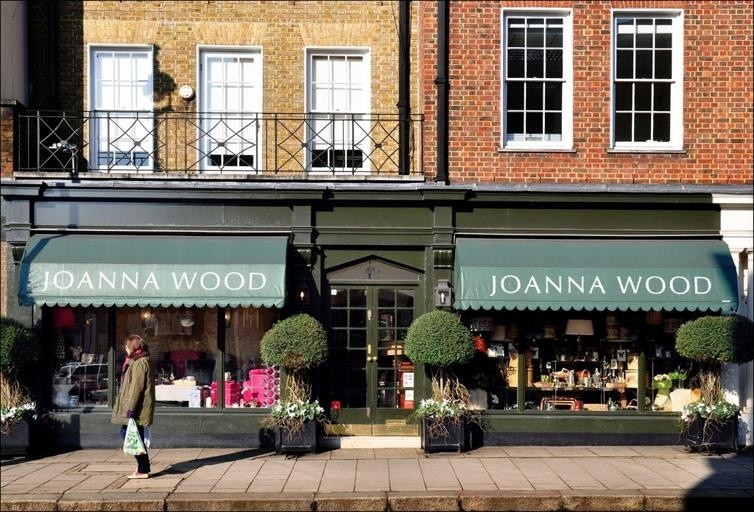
[541,383,614,411]
[155,384,202,409]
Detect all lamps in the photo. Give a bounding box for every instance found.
[564,320,595,359]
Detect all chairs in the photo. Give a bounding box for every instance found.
[242,369,277,409]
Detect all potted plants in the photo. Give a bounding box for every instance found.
[676,315,753,452]
[260,313,328,455]
[404,310,490,453]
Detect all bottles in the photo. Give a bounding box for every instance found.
[607,398,614,412]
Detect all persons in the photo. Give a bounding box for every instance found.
[111,335,155,479]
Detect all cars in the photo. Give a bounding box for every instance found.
[359,320,392,342]
[68,379,107,401]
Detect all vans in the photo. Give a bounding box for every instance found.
[55,364,107,385]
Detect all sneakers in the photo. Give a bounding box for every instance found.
[127,469,149,478]
